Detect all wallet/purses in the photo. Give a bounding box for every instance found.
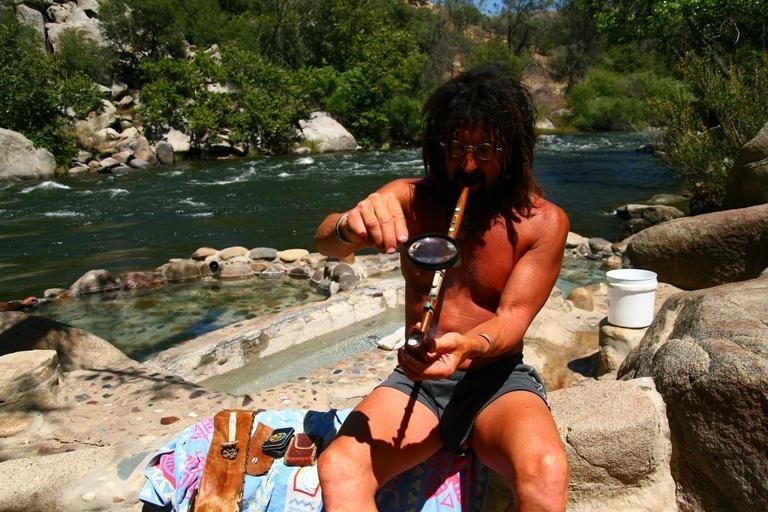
[262,428,295,458]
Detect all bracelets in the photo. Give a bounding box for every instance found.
[333,212,353,246]
[465,331,493,362]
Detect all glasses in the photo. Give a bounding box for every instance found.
[439,139,503,161]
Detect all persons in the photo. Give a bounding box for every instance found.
[311,63,576,512]
[0,294,43,314]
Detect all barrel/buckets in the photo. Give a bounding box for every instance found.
[605,268,658,329]
